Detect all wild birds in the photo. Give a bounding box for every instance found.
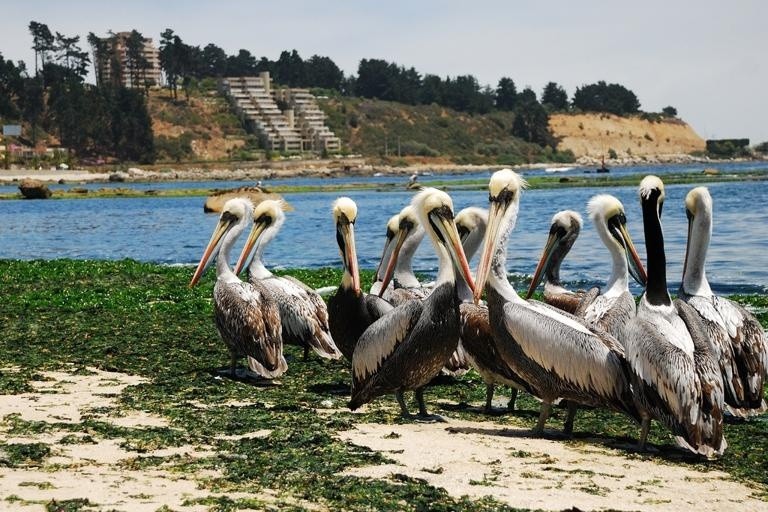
[189,167,768,461]
